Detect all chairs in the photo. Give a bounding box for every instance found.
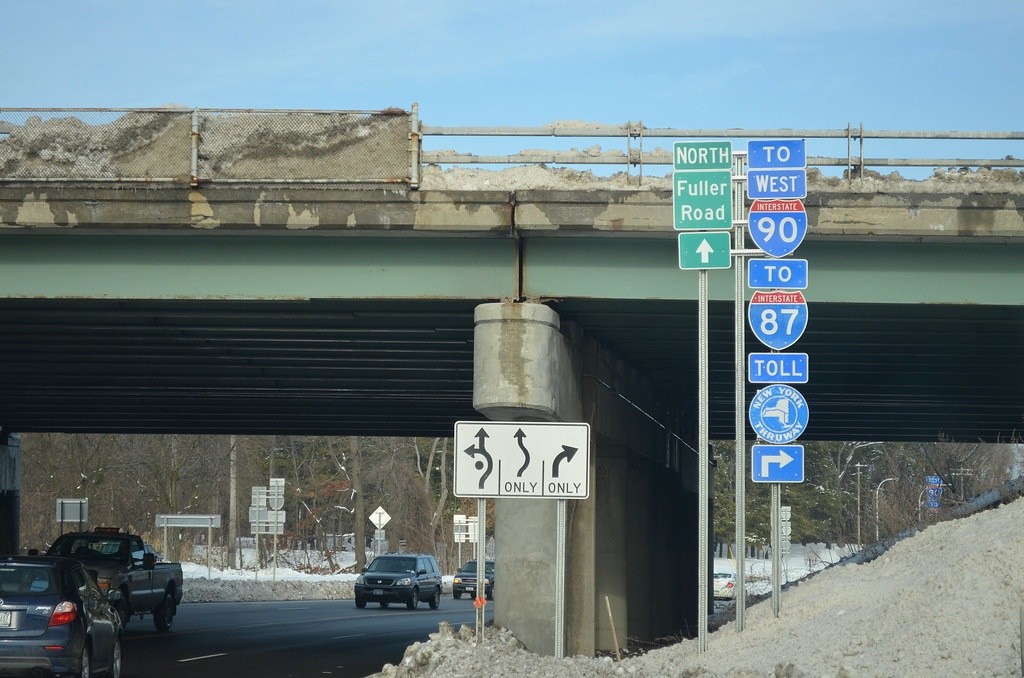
[76,546,89,554]
[1,583,20,591]
[30,580,49,592]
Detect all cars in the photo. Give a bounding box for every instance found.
[713,557,747,600]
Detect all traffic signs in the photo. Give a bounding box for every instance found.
[925,476,944,508]
[453,421,591,500]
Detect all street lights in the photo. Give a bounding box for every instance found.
[918,484,952,521]
[875,477,901,541]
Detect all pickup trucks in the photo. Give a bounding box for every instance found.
[42,530,184,635]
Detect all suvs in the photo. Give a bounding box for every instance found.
[452,559,495,600]
[354,552,442,610]
[0,554,122,678]
